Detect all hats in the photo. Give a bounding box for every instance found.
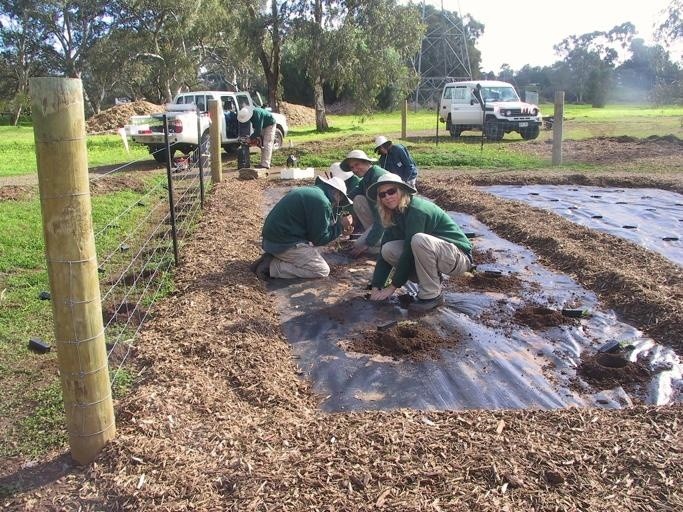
[312,176,353,208]
[365,172,416,204]
[373,135,393,153]
[330,150,378,179]
[237,105,255,123]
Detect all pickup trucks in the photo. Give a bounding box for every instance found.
[122,90,287,168]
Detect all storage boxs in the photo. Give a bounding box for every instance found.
[238,168,266,180]
[280,167,314,180]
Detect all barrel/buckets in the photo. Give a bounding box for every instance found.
[175,159,188,172]
[286,154,298,168]
[199,156,209,169]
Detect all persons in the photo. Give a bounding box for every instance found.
[237,106,276,170]
[374,136,418,187]
[366,174,473,313]
[251,174,353,279]
[340,150,394,257]
[331,161,360,192]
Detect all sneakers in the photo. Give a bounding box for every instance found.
[252,163,271,169]
[407,289,446,314]
[251,253,271,274]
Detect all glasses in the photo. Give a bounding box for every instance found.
[378,187,398,198]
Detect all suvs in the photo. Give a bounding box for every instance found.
[439,80,540,142]
